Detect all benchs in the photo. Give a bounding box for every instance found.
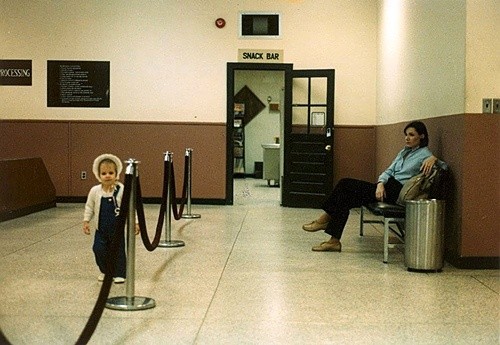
[358,199,406,262]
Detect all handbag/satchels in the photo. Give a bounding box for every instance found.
[396,167,442,206]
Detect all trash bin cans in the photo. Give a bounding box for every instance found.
[405,198,446,273]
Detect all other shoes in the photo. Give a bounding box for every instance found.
[312,241,341,251]
[303,220,330,232]
[97,273,105,281]
[112,277,125,283]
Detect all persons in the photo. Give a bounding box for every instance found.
[301,120,449,252]
[82,153,140,283]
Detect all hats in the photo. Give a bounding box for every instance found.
[92,153,123,182]
